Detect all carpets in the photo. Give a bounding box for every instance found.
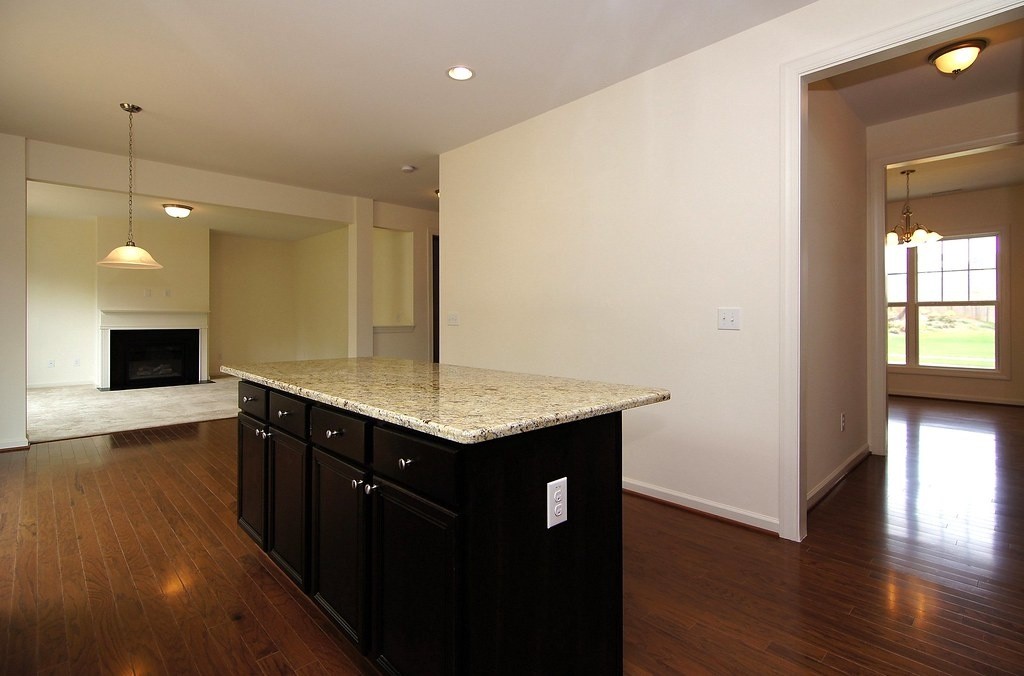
[26,375,242,444]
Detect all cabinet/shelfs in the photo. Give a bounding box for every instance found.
[309,401,623,675]
[236,377,309,593]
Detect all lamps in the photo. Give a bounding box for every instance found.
[885,169,944,253]
[97,102,164,269]
[928,39,987,75]
[162,204,193,218]
